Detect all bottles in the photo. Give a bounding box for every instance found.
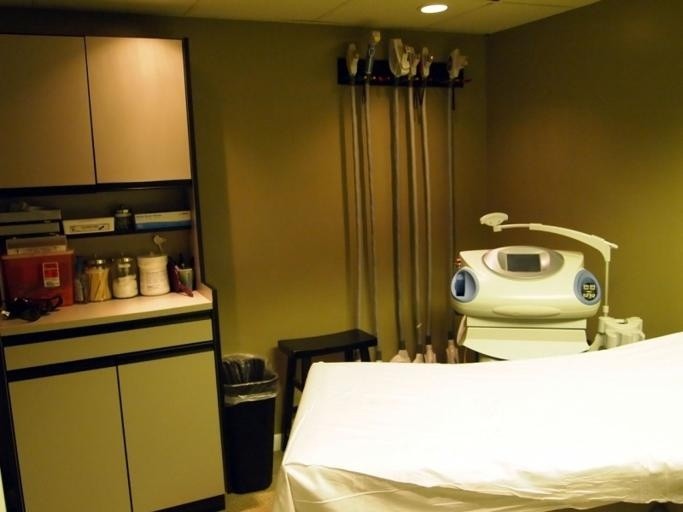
[73,251,138,302]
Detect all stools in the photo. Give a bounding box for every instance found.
[277,329,377,451]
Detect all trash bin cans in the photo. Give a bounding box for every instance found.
[221,353,279,494]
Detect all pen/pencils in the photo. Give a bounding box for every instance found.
[168,253,185,270]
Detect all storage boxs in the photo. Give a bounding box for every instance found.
[1,236,74,310]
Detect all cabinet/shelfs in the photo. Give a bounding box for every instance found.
[0,34,225,510]
